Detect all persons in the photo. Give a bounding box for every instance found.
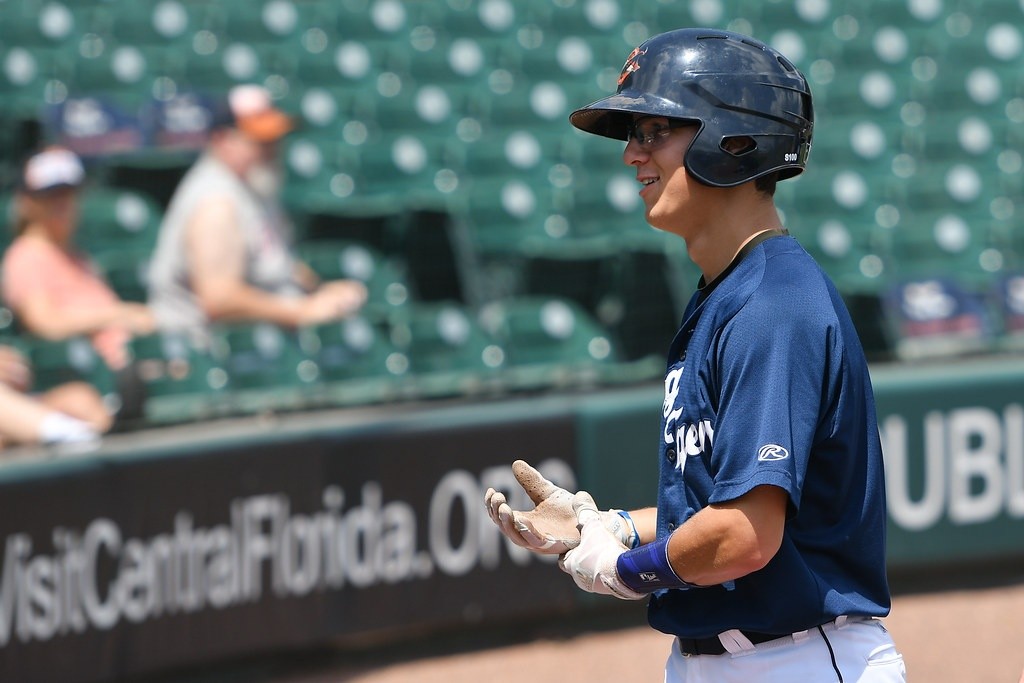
[147,83,368,329]
[0,150,210,449]
[485,27,907,683]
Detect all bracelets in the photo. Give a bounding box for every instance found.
[615,509,640,550]
[615,528,709,595]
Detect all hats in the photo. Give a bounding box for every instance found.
[26,150,82,190]
[219,84,293,142]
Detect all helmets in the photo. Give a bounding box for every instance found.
[569,28,814,188]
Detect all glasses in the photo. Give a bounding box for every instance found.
[628,116,702,148]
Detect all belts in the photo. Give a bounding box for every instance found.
[680,627,820,654]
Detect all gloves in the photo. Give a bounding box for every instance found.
[558,491,711,601]
[484,460,640,553]
